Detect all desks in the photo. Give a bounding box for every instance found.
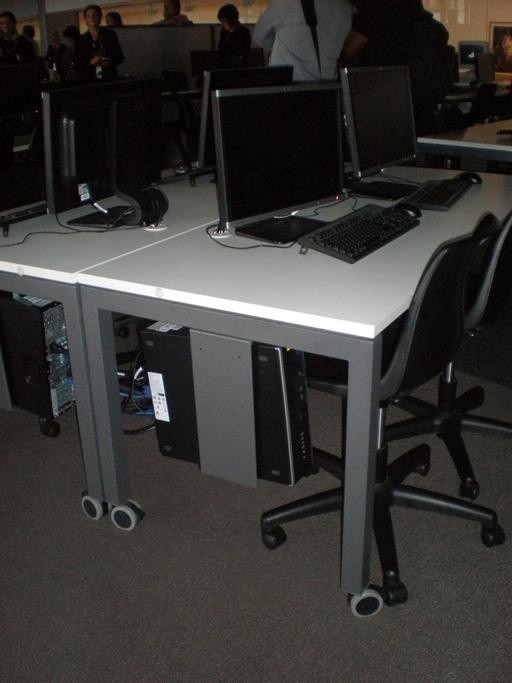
[417,118,512,174]
[77,163,512,621]
[1,167,222,522]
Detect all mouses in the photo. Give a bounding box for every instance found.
[394,203,421,218]
[455,173,481,184]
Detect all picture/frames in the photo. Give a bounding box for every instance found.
[457,41,488,72]
[488,21,512,77]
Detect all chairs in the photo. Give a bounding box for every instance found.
[0,123,46,223]
[28,123,45,167]
[259,209,505,608]
[380,212,512,501]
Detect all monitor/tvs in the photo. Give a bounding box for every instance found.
[196,65,294,185]
[0,63,38,136]
[41,77,169,228]
[457,41,488,80]
[339,65,419,201]
[211,83,345,244]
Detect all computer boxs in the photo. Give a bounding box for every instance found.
[0,290,77,437]
[139,322,314,488]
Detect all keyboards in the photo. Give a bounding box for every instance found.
[397,176,471,212]
[0,203,46,228]
[296,204,420,264]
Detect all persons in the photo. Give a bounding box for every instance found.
[1,1,190,85]
[216,1,459,147]
